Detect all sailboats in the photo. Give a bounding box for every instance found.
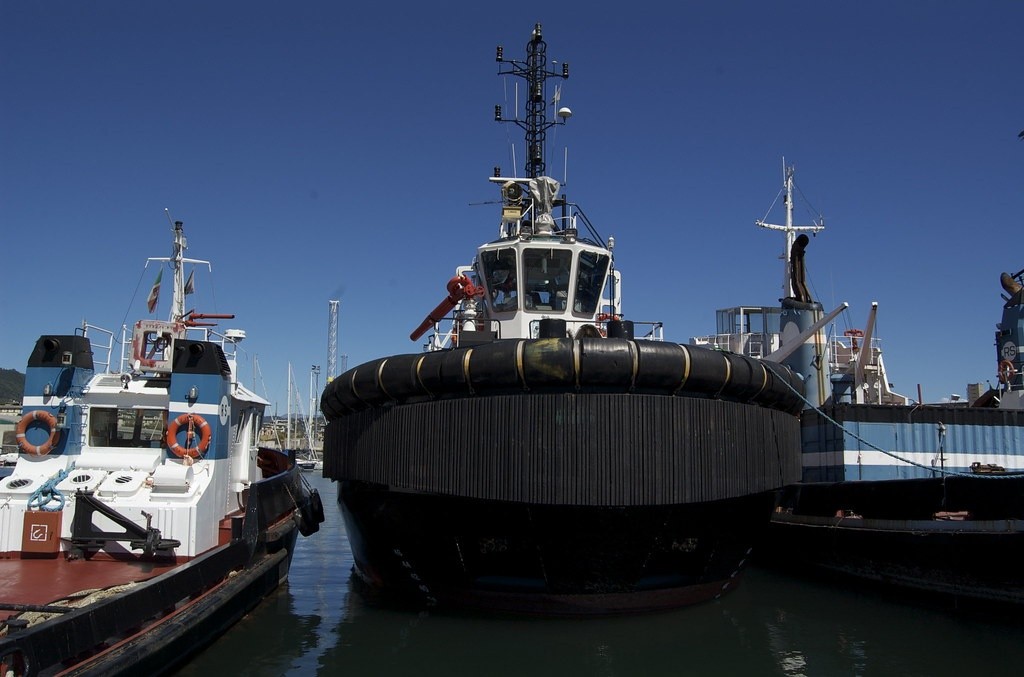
[252,353,324,472]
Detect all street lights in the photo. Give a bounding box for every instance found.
[312,364,321,447]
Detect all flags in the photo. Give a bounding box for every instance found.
[147,267,163,314]
[184,270,195,296]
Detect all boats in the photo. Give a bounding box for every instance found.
[323,21,1024,640]
[0,206,326,677]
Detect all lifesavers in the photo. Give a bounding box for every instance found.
[166,415,211,458]
[596,314,620,337]
[998,361,1014,382]
[15,409,60,458]
